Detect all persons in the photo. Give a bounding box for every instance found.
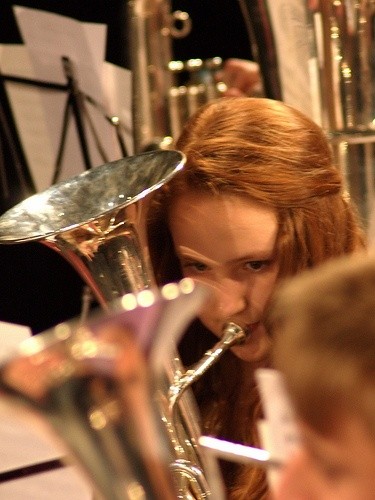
[265,243,373,500]
[143,93,366,499]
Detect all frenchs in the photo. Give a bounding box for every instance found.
[0,148,244,492]
[0,278,215,500]
